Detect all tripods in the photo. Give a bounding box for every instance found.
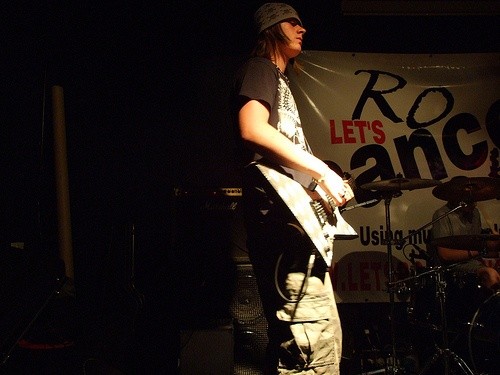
[387,240,488,375]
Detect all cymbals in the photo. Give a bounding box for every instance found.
[432,177,500,202]
[360,177,443,191]
[425,235,500,251]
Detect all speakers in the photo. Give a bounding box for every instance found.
[169,185,268,375]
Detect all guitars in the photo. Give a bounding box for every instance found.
[255,162,360,269]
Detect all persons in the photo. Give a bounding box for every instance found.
[425,176,499,290]
[235,3,356,375]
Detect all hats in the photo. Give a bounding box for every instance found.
[255,3,303,34]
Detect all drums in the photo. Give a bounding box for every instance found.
[447,292,500,375]
[406,267,477,333]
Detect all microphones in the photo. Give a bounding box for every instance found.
[413,244,433,261]
